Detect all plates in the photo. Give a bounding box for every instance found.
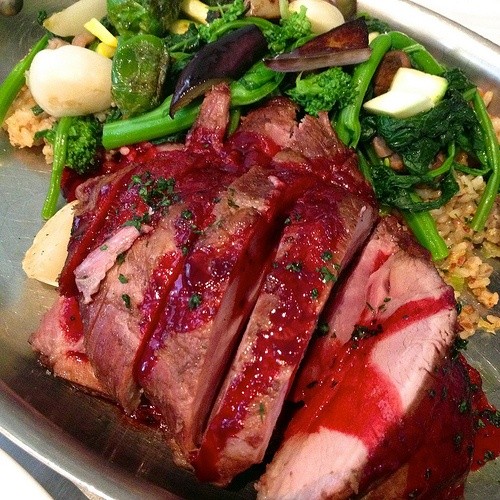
[0,0,500,500]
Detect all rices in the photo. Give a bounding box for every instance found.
[0,52,500,343]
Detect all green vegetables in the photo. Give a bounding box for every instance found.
[0,0,500,261]
